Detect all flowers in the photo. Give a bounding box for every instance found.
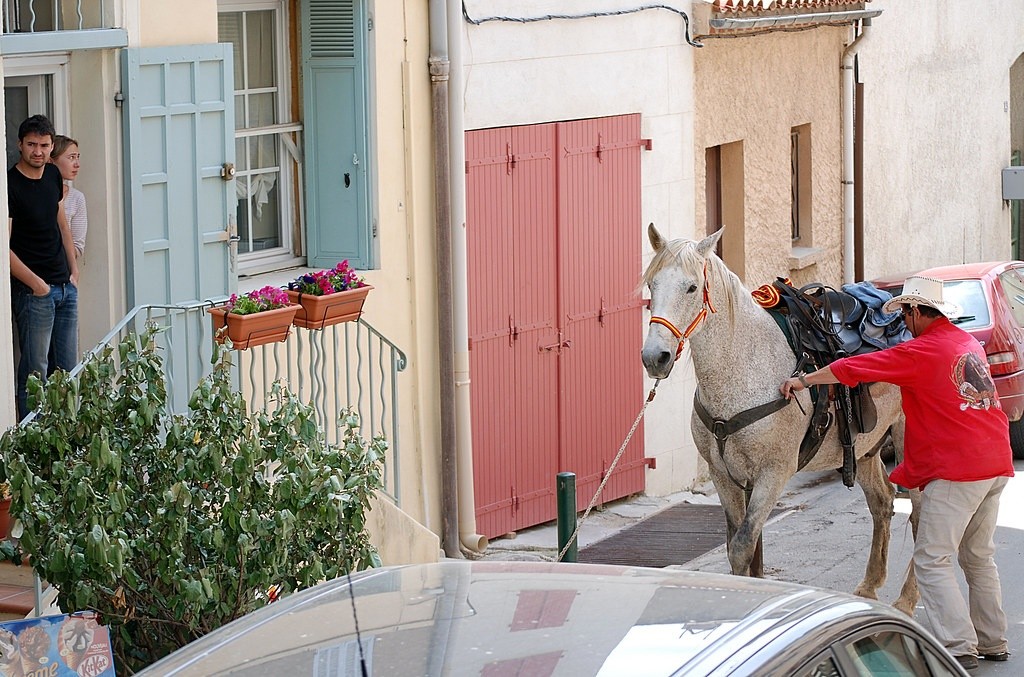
[227,284,290,315]
[281,258,366,296]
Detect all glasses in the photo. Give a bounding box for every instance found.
[901,309,912,321]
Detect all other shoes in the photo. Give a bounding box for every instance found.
[954,655,978,669]
[978,651,1011,660]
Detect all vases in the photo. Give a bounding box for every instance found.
[207,302,302,350]
[283,284,375,330]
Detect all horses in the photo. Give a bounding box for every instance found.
[629,223,924,618]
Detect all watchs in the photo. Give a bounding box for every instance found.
[798,373,812,388]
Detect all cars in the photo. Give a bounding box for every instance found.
[130,559,968,677]
[868,260,1024,461]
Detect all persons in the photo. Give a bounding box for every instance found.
[46,134,87,260]
[779,277,1015,671]
[4,115,79,422]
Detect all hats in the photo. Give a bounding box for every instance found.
[882,275,964,318]
